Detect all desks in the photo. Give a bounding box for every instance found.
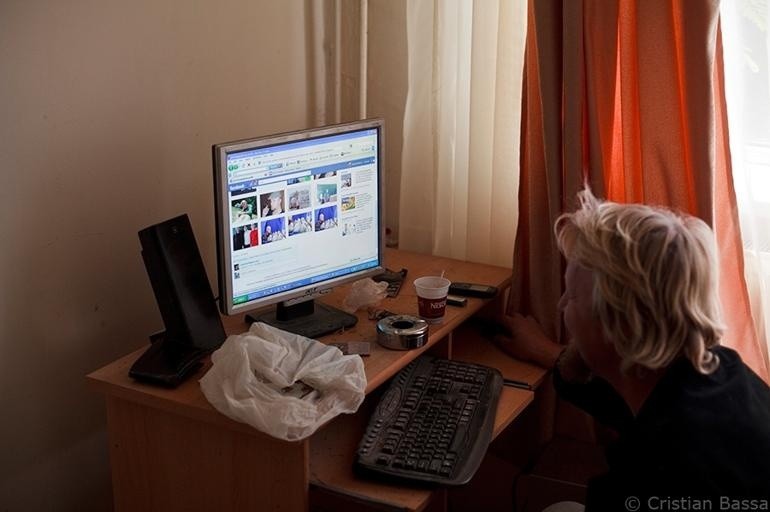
[87,248,549,512]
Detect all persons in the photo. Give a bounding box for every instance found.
[233,174,355,251]
[490,172,769,511]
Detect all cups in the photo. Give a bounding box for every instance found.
[414,276,450,323]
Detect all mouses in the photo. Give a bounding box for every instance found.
[478,321,511,338]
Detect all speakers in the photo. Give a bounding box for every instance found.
[137,212,228,356]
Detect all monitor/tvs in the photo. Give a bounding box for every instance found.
[211,116,387,339]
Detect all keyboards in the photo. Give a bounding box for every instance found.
[350,352,504,491]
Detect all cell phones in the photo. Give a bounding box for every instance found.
[448,282,498,299]
